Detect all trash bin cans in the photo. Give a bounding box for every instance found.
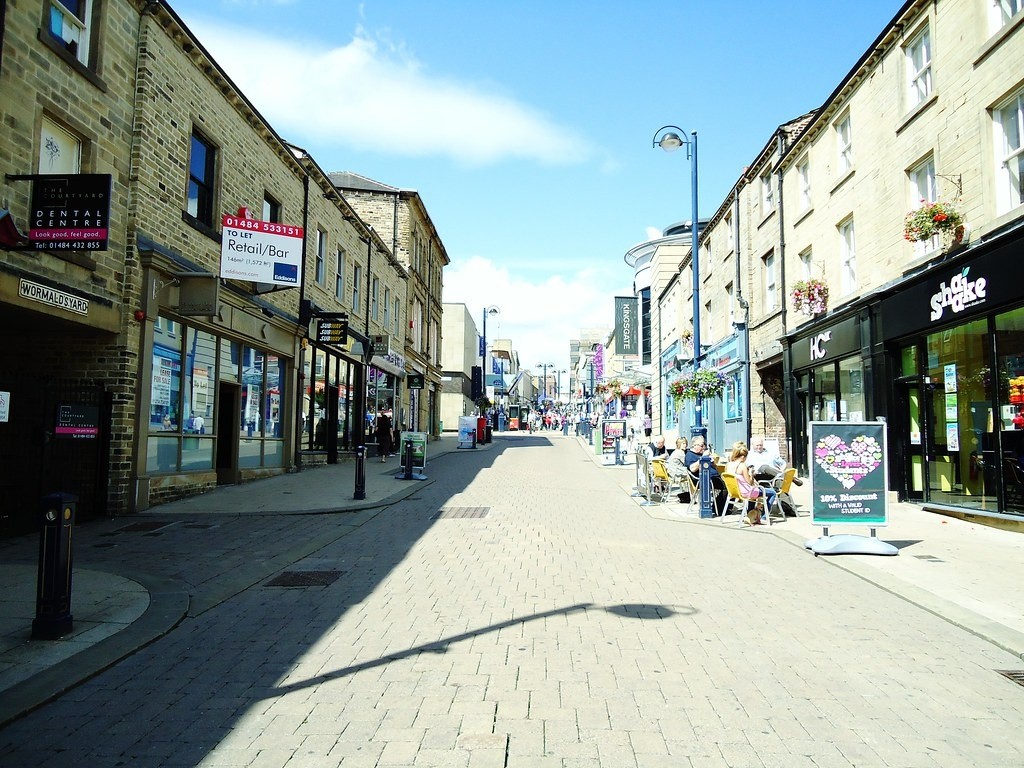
[486,425,494,443]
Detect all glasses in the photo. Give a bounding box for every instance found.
[695,443,704,447]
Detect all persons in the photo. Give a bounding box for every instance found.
[375,415,393,463]
[617,405,654,451]
[470,406,617,436]
[299,415,327,453]
[644,430,803,525]
[187,410,204,434]
[163,415,174,431]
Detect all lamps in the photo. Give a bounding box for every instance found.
[300,337,308,351]
[342,216,358,223]
[398,274,407,279]
[377,249,387,253]
[359,237,369,243]
[242,305,275,319]
[388,260,399,267]
[323,193,338,202]
[350,342,364,355]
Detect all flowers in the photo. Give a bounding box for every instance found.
[596,379,623,399]
[667,368,729,400]
[903,198,964,243]
[681,330,694,349]
[790,279,830,313]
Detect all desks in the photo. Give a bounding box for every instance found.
[716,464,725,475]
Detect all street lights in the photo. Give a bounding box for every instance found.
[553,369,567,400]
[536,362,555,399]
[483,305,501,397]
[653,125,708,450]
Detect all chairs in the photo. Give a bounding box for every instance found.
[650,457,719,516]
[721,472,772,528]
[758,467,802,519]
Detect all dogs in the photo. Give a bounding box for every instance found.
[744,503,764,525]
